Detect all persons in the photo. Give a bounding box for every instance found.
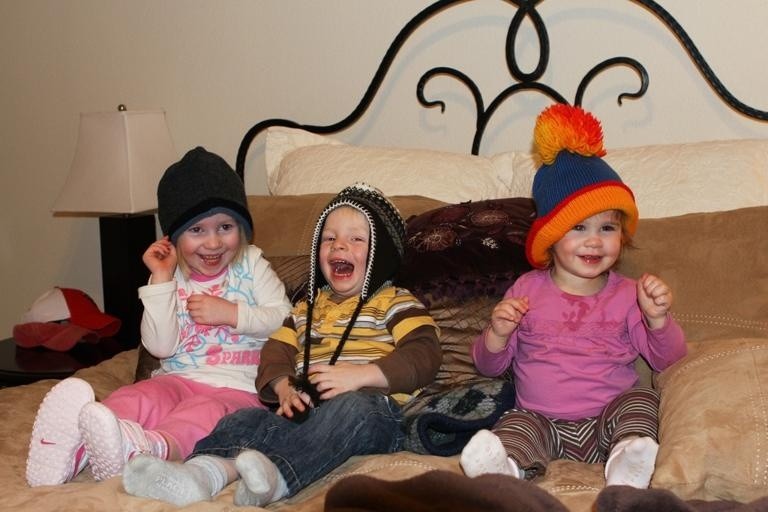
[122,182,443,507]
[25,146,293,488]
[459,210,688,489]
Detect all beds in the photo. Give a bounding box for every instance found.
[1,0,767,511]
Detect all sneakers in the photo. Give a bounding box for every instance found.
[25,375,168,489]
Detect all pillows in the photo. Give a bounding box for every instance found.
[613,203,763,329]
[490,136,762,223]
[263,128,518,206]
[247,194,456,258]
[648,335,765,511]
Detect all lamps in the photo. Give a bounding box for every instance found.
[51,104,181,314]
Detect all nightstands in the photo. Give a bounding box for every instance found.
[1,337,142,386]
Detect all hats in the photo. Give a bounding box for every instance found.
[524,104,638,270]
[156,146,254,244]
[308,180,408,302]
[9,286,120,353]
